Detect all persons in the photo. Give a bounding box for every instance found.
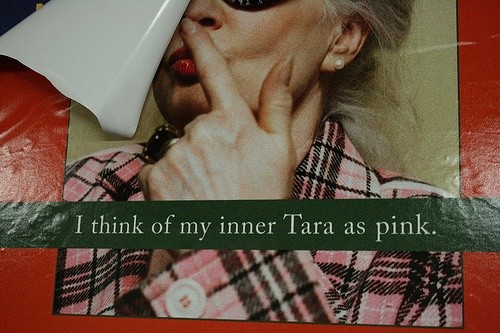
[53,1,465,329]
